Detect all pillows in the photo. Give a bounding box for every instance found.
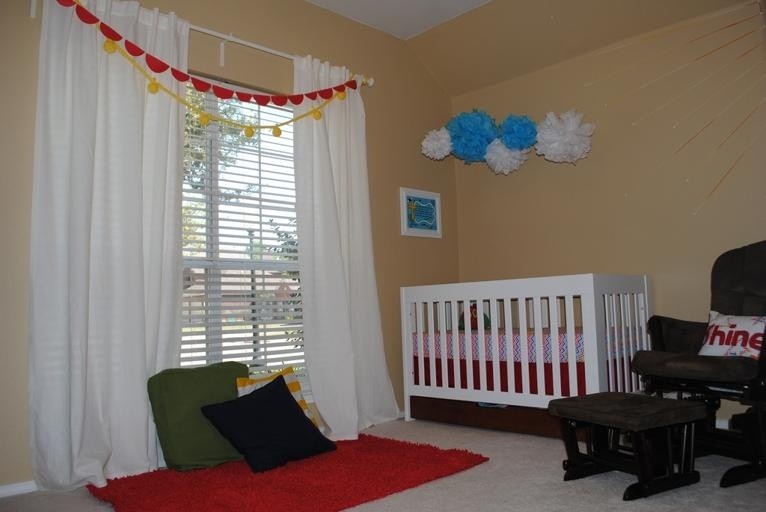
[148,361,249,473]
[201,375,337,474]
[249,369,332,437]
[236,367,318,427]
[697,310,766,361]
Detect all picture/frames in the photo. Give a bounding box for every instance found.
[399,186,442,238]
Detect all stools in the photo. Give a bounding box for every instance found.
[547,394,713,503]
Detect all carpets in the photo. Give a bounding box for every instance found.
[86,427,489,512]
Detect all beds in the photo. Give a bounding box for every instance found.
[398,273,650,445]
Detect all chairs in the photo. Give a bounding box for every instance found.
[631,240,766,488]
